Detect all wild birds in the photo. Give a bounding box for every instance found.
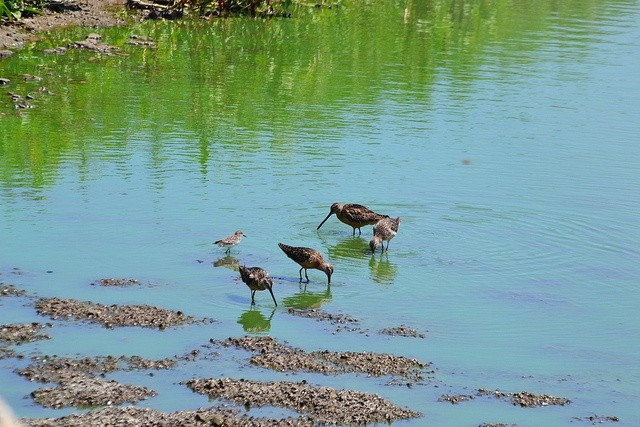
[369,215,401,256]
[211,229,247,253]
[277,241,334,285]
[316,202,388,236]
[239,264,279,307]
